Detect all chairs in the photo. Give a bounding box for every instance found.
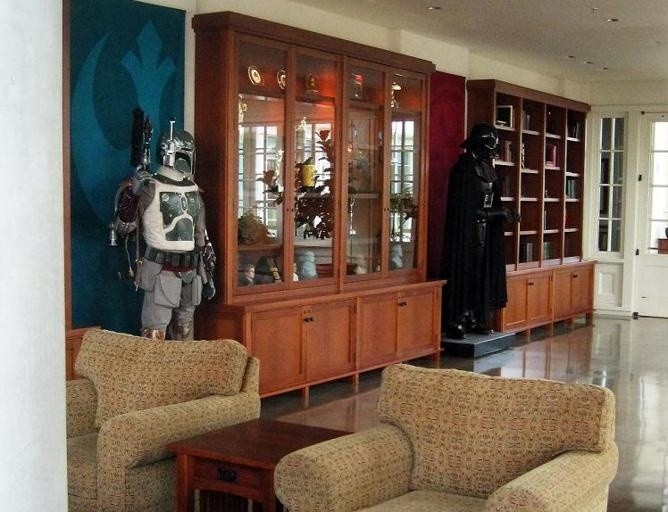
[274,362,620,512]
[66,327,261,512]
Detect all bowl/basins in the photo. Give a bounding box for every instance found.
[316,263,356,278]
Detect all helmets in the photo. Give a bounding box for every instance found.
[160,129,197,169]
[458,122,502,157]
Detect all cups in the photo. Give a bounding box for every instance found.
[301,165,316,187]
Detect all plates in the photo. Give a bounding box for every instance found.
[247,64,287,90]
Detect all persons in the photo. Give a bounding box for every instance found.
[112,122,215,339]
[436,119,515,338]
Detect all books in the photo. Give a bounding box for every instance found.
[519,241,555,263]
[490,136,515,198]
[564,178,579,200]
[543,141,563,167]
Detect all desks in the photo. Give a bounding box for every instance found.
[166,418,355,512]
